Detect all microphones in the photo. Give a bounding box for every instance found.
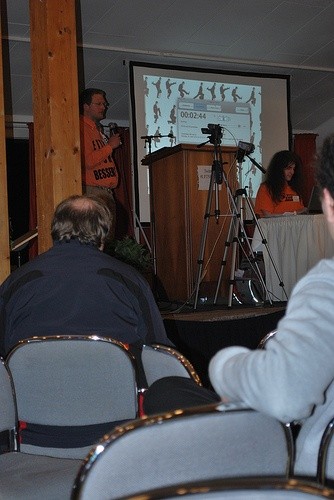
[109,123,122,144]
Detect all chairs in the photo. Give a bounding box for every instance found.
[0,334,334,500]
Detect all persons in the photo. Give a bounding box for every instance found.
[79,87,122,195]
[143,133,334,480]
[1,196,177,388]
[254,150,306,217]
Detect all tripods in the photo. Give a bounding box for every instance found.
[191,141,289,309]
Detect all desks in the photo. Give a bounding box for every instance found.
[251,212,334,301]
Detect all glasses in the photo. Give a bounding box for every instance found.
[87,101,107,107]
